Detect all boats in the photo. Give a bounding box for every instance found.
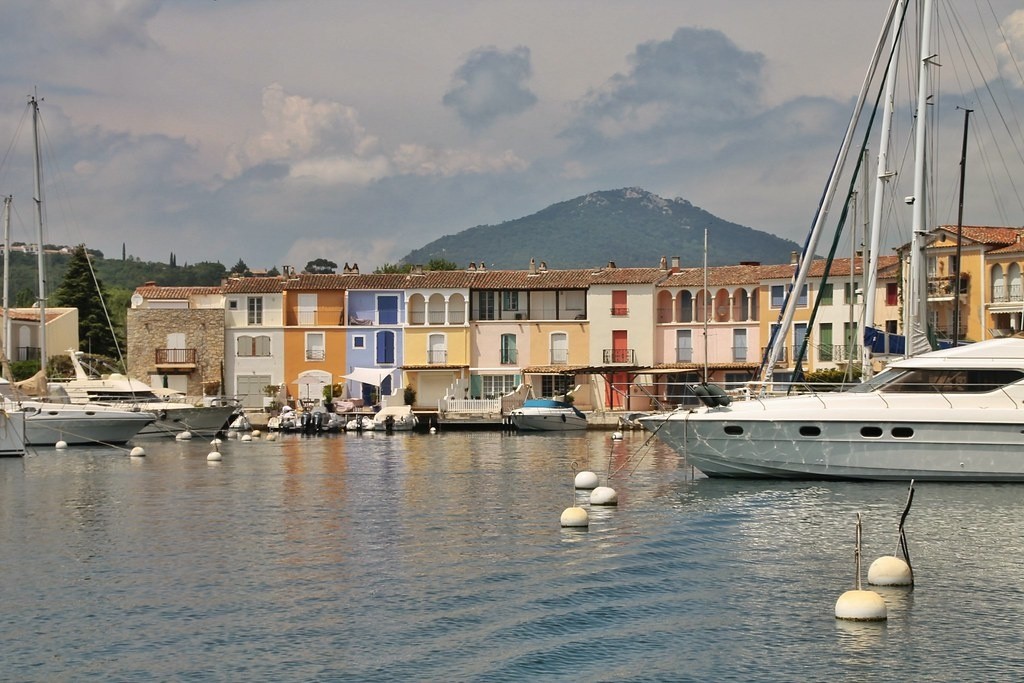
[219,414,239,433]
[321,413,346,430]
[509,399,587,430]
[46,347,153,400]
[267,415,303,429]
[229,416,247,431]
[374,405,415,430]
[346,416,375,430]
[0,408,26,455]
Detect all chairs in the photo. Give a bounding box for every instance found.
[300,399,321,412]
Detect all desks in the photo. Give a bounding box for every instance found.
[303,400,314,406]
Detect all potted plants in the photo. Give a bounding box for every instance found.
[261,384,280,413]
[370,392,381,413]
[204,379,221,407]
[268,401,279,418]
[322,384,335,412]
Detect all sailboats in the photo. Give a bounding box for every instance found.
[0,193,165,444]
[610,0,1023,482]
[0,84,245,440]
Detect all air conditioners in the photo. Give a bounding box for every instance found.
[513,312,526,320]
